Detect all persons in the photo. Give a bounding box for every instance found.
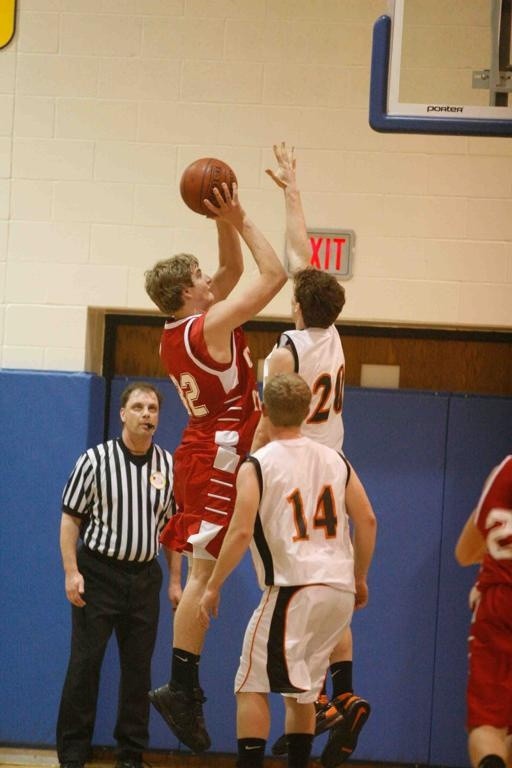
[450,455,511,768]
[57,382,183,768]
[145,182,288,754]
[246,140,373,767]
[197,375,378,768]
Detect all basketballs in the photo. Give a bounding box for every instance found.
[180,157,238,215]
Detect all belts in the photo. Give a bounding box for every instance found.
[92,549,158,570]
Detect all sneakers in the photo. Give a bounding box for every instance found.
[272,697,341,754]
[320,695,371,767]
[150,682,212,752]
